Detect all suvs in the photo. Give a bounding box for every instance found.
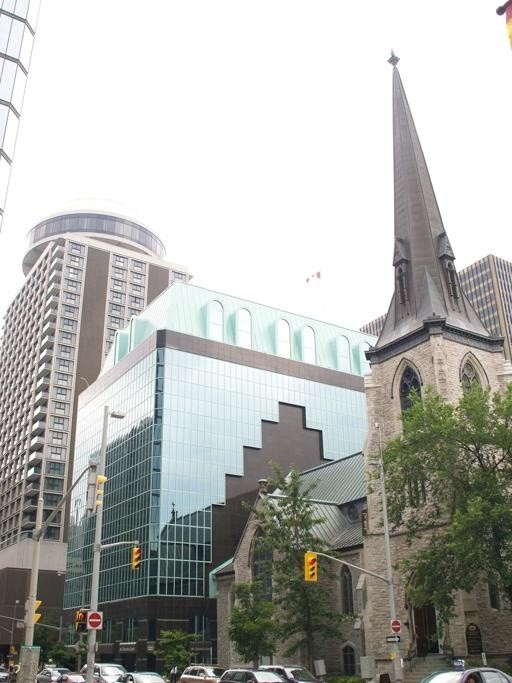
[81,662,125,683]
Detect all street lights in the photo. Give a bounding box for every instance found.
[84,406,125,664]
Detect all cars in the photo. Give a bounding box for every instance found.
[0,666,8,681]
[219,665,291,683]
[36,666,107,683]
[258,662,323,683]
[118,671,164,683]
[180,662,228,682]
[420,658,512,683]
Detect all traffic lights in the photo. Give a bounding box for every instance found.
[95,473,107,506]
[34,600,41,624]
[304,551,318,582]
[132,547,141,570]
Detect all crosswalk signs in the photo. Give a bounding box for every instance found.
[75,610,86,631]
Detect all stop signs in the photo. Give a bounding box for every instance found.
[87,611,103,630]
[391,620,401,633]
